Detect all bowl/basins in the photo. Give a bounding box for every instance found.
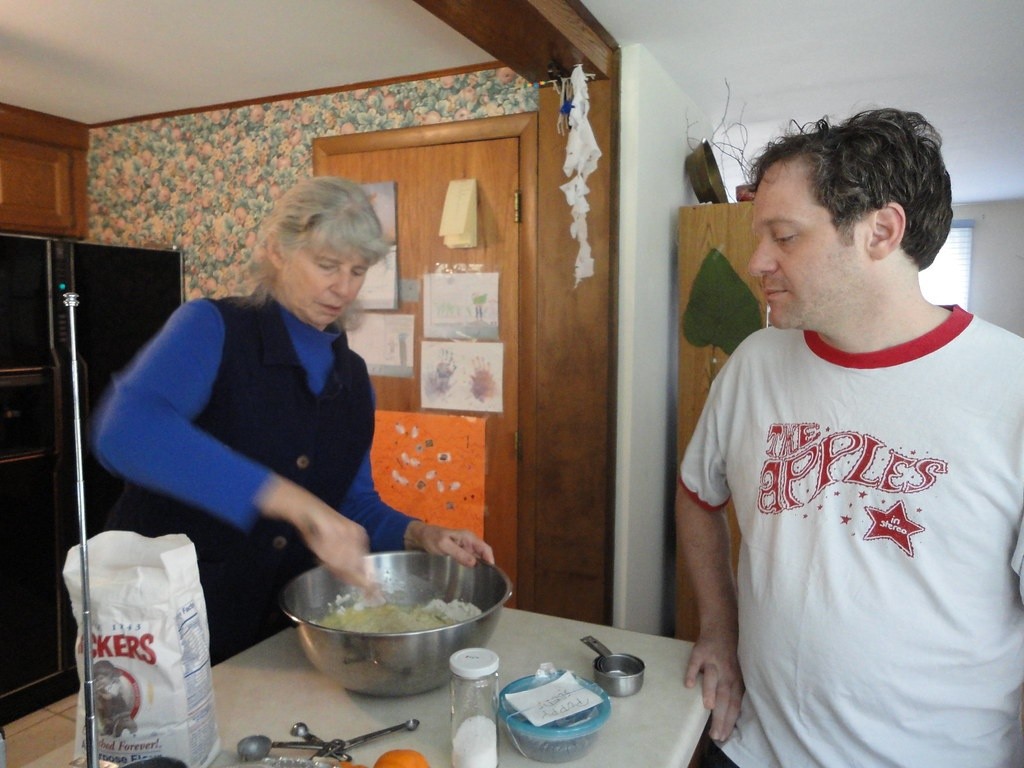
[279,551,515,700]
[498,673,612,763]
[580,636,645,698]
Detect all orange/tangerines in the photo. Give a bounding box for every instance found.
[372,749,429,768]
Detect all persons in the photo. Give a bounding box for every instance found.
[676,109,1024,768]
[92,177,496,666]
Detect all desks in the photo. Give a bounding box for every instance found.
[19,607,718,768]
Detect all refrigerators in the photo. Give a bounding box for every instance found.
[0,233,184,727]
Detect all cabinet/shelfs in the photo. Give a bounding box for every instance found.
[677,203,769,643]
[0,233,184,730]
[0,102,92,239]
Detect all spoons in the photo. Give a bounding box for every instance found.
[237,718,419,761]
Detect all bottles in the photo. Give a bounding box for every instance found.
[449,646,500,768]
